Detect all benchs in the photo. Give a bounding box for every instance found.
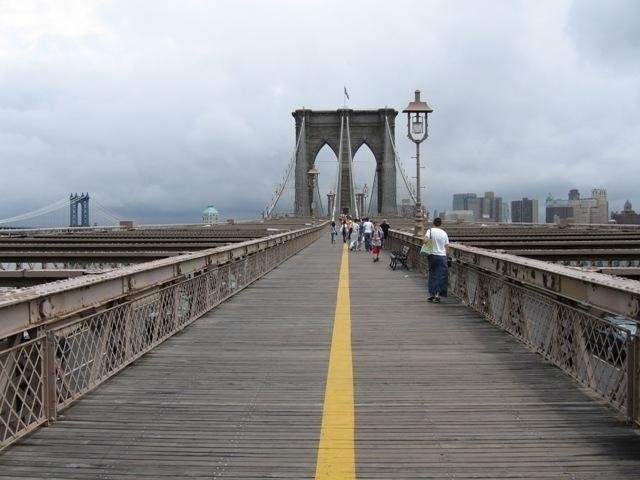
[387,244,411,271]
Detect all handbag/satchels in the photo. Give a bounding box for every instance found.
[420,228,433,257]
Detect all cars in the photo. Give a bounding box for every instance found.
[12,331,70,375]
[591,314,638,364]
[144,310,184,334]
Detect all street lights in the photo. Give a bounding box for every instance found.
[363,184,369,218]
[306,166,320,226]
[401,88,435,239]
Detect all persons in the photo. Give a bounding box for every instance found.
[339,214,390,263]
[329,221,337,244]
[423,218,450,303]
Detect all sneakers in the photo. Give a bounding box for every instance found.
[435,296,441,304]
[428,297,435,301]
